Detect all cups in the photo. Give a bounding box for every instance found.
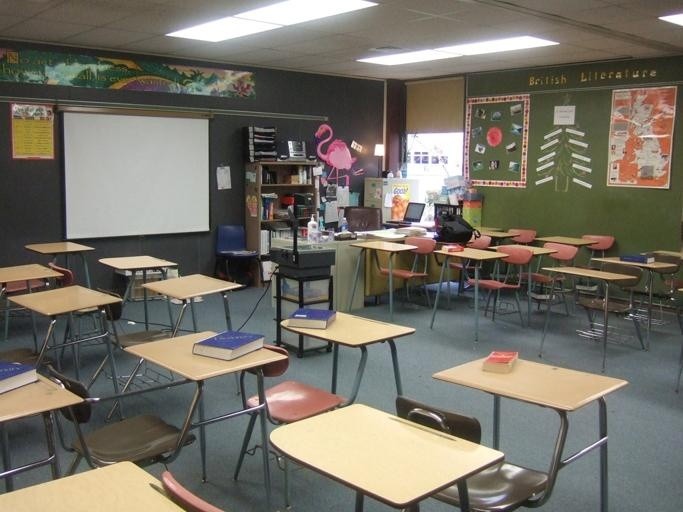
[309,231,322,244]
[328,228,334,241]
[386,173,392,178]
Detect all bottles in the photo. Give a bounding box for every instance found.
[340,217,347,231]
[401,160,408,178]
[265,202,273,220]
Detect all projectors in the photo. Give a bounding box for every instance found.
[268,243,336,267]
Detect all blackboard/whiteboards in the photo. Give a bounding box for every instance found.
[467,54,683,306]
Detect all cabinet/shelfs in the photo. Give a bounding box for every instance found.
[243,160,320,289]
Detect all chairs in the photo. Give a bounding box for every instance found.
[344,206,381,234]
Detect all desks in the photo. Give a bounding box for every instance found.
[1,223,680,511]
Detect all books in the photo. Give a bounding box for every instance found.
[192,330,265,360]
[442,245,464,252]
[482,351,518,373]
[287,308,336,329]
[261,166,313,255]
[620,256,655,264]
[0,362,39,394]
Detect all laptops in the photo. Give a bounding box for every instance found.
[386,202,426,224]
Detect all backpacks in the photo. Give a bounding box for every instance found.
[433,210,480,246]
